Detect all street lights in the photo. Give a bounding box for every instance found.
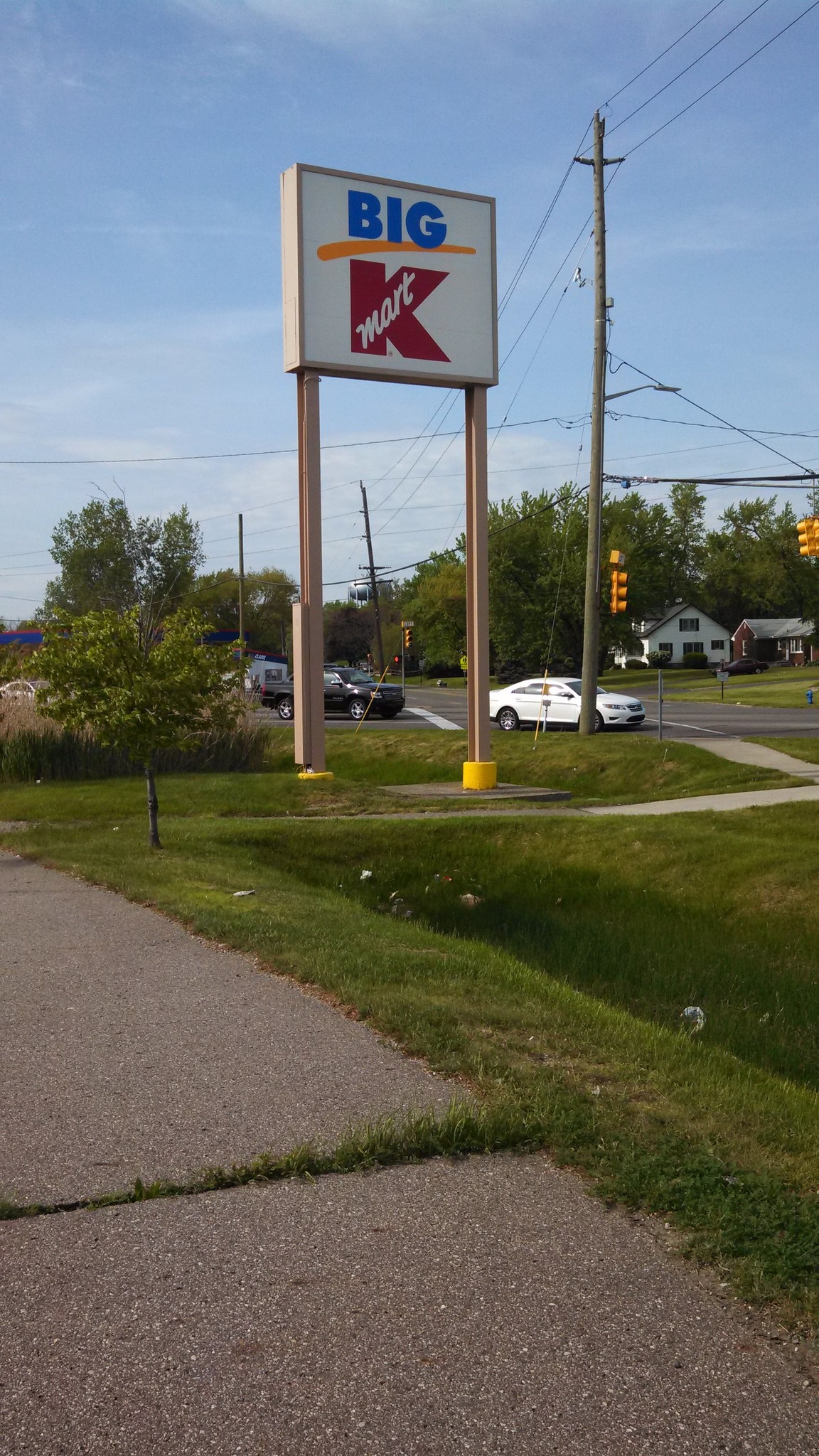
[580,386,684,734]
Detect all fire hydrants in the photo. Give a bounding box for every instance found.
[805,690,813,704]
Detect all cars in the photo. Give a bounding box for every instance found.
[489,677,646,733]
[715,658,770,677]
[359,662,373,672]
[261,663,406,721]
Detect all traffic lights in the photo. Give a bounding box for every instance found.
[394,655,402,663]
[406,655,415,662]
[796,519,819,557]
[611,570,628,614]
[406,629,413,648]
